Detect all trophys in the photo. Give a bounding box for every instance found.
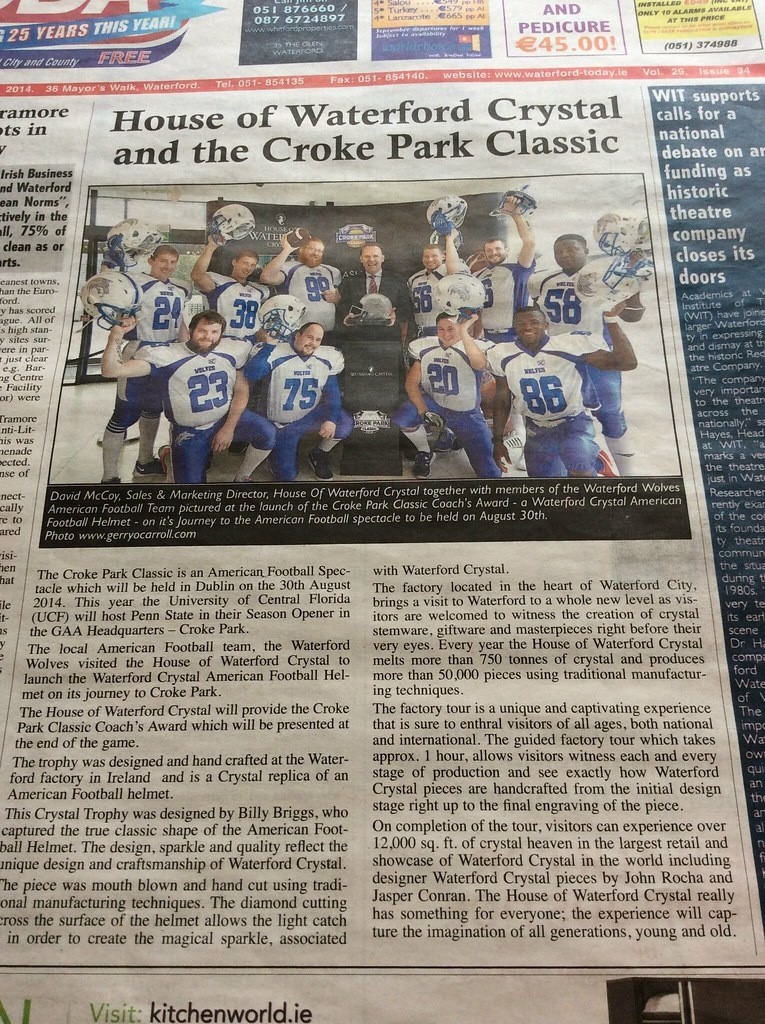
[358,294,393,326]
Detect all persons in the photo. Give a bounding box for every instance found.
[260,232,342,342]
[338,241,416,461]
[518,233,648,470]
[406,228,471,452]
[485,301,638,479]
[101,311,277,483]
[395,314,508,478]
[243,332,353,482]
[102,245,194,482]
[470,196,534,344]
[190,234,274,472]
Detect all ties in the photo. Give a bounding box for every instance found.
[368,275,377,295]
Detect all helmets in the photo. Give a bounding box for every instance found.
[79,191,650,339]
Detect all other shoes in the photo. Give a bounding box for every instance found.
[97,413,614,478]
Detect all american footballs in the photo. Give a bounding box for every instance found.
[287,226,310,244]
